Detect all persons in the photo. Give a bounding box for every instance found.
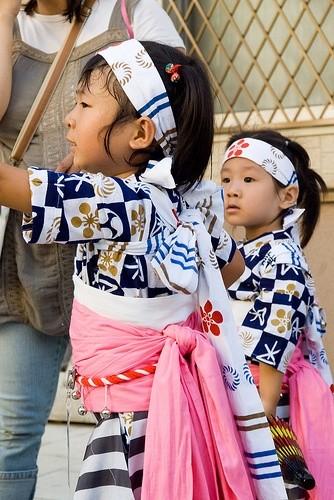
[0,0,187,498]
[214,125,333,498]
[0,41,288,499]
